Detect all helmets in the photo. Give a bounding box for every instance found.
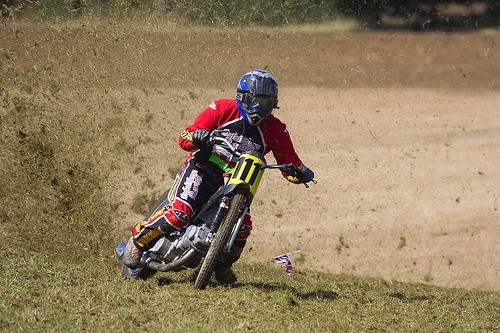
[235,69,280,126]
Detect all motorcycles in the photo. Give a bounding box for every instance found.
[115,129,318,290]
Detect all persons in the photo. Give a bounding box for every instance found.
[121,67,315,286]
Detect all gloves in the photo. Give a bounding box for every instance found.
[192,129,210,148]
[294,165,315,183]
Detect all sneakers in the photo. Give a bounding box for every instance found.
[121,236,143,269]
[214,266,236,284]
